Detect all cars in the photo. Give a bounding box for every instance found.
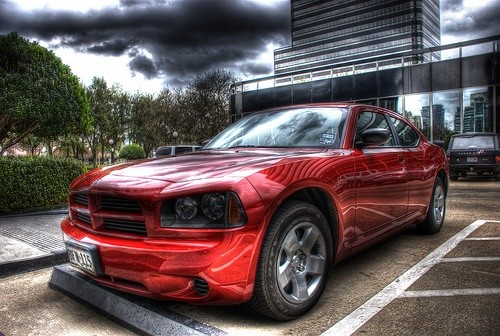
[60,103,448,323]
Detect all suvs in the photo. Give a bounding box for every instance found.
[447,132,500,180]
